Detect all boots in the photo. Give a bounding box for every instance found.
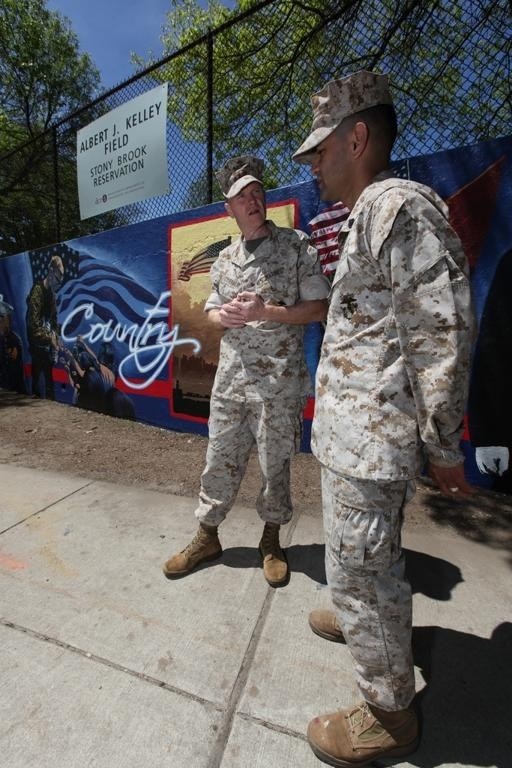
[163,520,224,579]
[256,521,290,587]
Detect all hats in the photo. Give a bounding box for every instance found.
[212,156,266,197]
[291,69,395,164]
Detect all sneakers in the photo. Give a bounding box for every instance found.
[310,608,350,645]
[308,700,421,767]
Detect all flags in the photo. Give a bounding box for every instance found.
[438,151,508,262]
[28,242,169,353]
[177,235,233,283]
[305,157,412,279]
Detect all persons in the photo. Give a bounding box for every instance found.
[161,153,330,590]
[285,63,476,768]
[54,326,137,423]
[468,248,512,495]
[22,252,69,400]
[0,291,27,395]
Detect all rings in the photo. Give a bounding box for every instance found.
[449,487,459,493]
